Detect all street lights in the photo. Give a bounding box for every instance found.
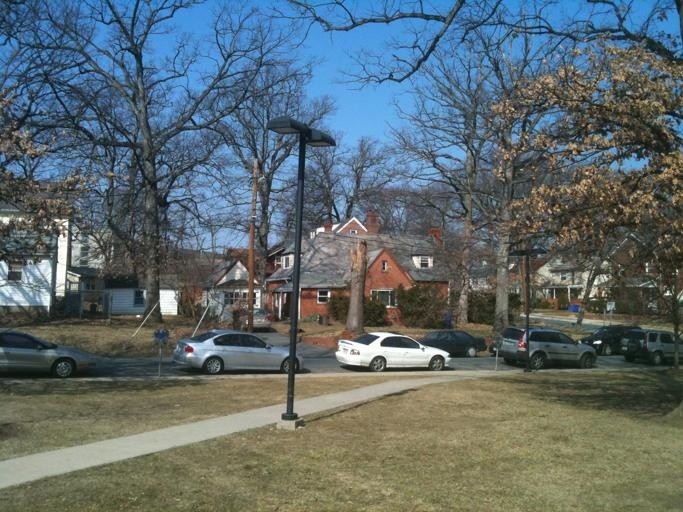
[509,247,545,374]
[264,114,335,423]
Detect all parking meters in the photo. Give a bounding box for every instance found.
[151,327,169,378]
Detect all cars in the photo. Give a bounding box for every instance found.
[488,318,682,369]
[335,329,450,373]
[0,328,103,377]
[648,295,673,315]
[417,327,485,357]
[172,331,303,374]
[240,306,273,330]
